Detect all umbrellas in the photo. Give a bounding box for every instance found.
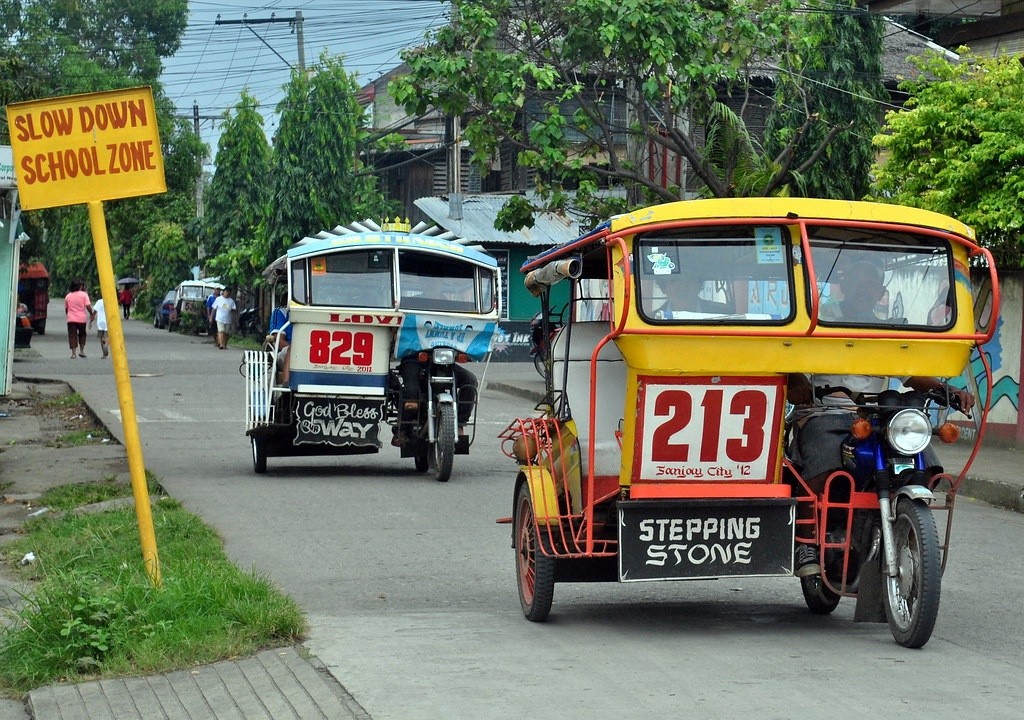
[117,277,139,284]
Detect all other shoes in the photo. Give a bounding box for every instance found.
[458,426,464,435]
[79,353,86,357]
[103,347,108,356]
[392,432,405,445]
[219,346,225,349]
[71,354,77,359]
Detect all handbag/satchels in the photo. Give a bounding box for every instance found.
[118,293,125,304]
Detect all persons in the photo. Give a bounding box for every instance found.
[412,265,448,301]
[649,249,735,320]
[786,257,975,577]
[209,287,237,350]
[120,284,134,319]
[206,285,222,347]
[272,293,294,387]
[64,279,94,359]
[89,298,111,359]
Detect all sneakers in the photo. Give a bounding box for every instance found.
[794,544,821,577]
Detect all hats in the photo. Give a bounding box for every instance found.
[848,253,886,280]
[214,284,221,289]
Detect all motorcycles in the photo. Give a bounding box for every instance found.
[244,305,263,335]
[244,216,503,481]
[169,280,226,332]
[496,199,1000,648]
[239,300,253,336]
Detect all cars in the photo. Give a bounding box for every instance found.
[153,291,176,329]
